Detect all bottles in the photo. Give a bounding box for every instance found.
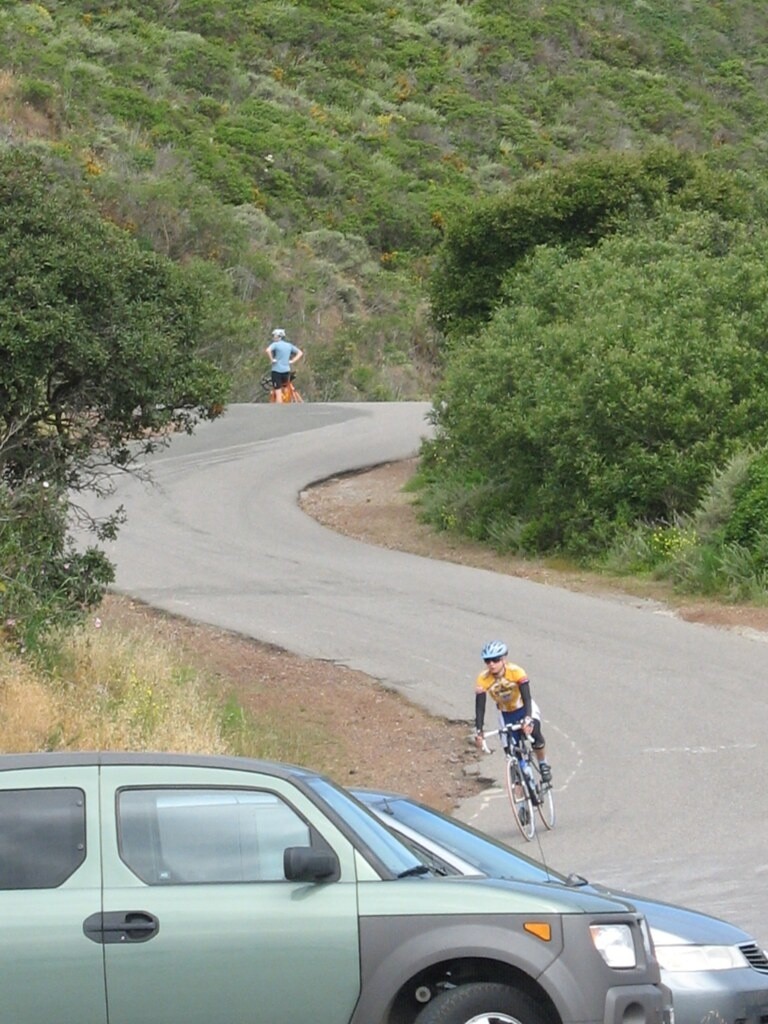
[521,760,537,790]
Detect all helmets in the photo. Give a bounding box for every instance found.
[272,329,285,337]
[480,640,507,660]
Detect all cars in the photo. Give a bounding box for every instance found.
[1,753,676,1024]
[153,787,768,1024]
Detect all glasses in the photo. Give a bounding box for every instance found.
[484,657,500,665]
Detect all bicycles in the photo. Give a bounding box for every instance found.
[467,716,555,842]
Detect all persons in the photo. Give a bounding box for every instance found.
[475,642,552,825]
[266,328,304,404]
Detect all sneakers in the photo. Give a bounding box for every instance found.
[539,764,552,782]
[518,807,528,826]
